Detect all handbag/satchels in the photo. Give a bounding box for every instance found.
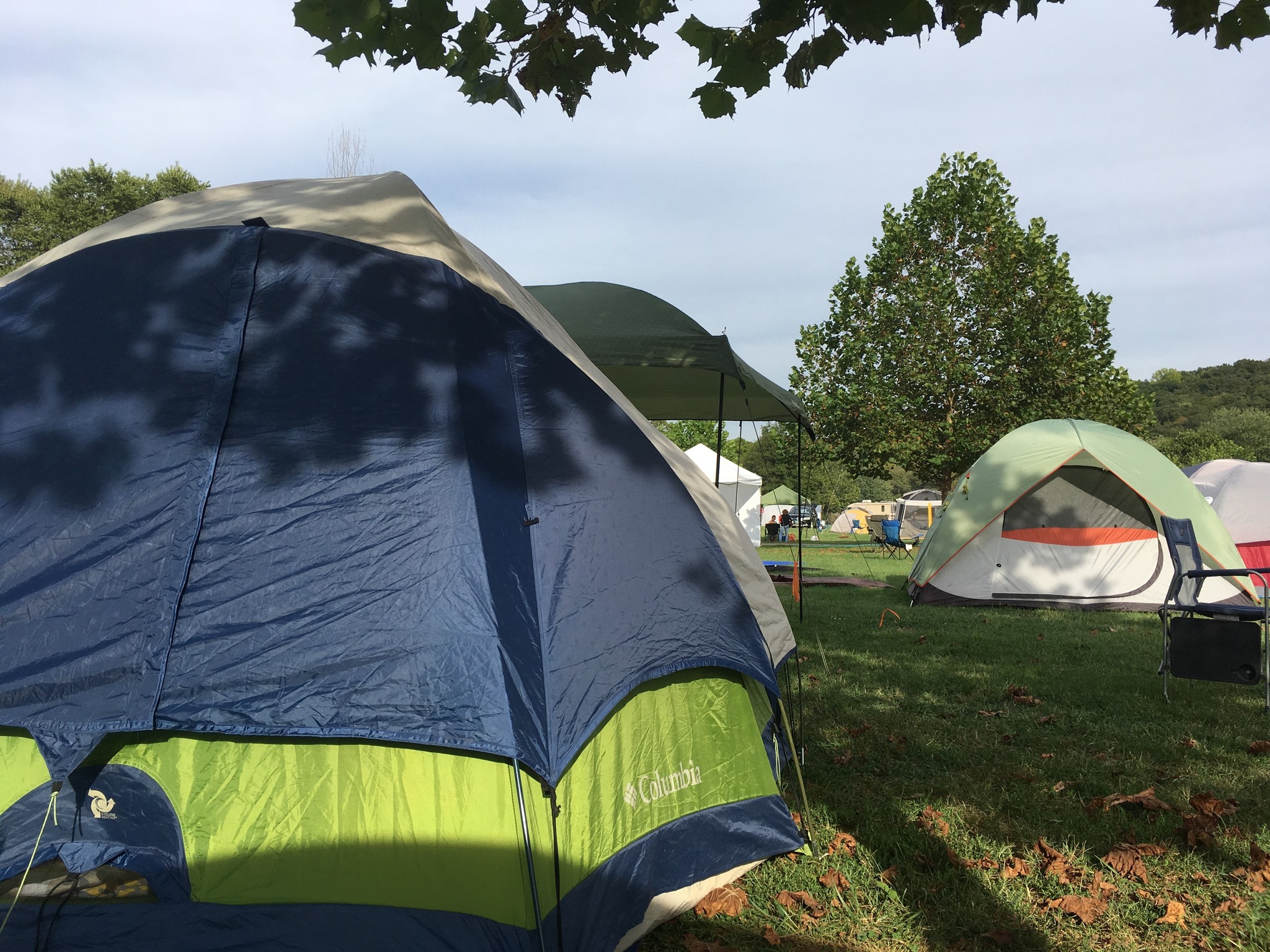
[789,521,793,526]
[788,534,796,541]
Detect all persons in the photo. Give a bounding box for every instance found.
[768,515,777,524]
[779,509,791,542]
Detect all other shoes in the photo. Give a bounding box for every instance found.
[769,539,771,542]
[773,538,776,541]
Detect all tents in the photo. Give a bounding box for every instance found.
[0,173,816,952]
[824,508,874,535]
[895,419,1270,610]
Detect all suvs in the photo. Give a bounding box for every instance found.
[788,506,818,529]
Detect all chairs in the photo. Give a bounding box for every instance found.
[816,518,829,534]
[765,523,780,542]
[1158,515,1270,703]
[851,515,924,561]
